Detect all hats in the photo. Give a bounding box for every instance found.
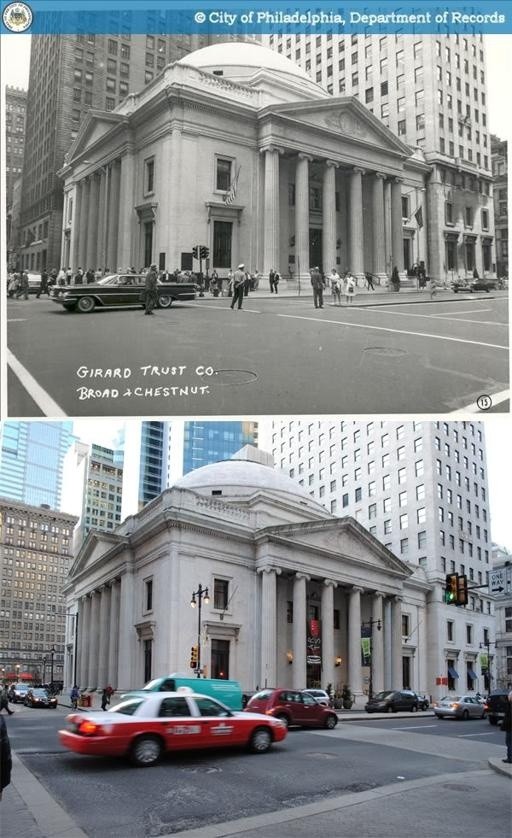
[238,263,244,268]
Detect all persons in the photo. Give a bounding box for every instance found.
[70,685,81,709]
[0,684,15,715]
[273,271,279,293]
[329,268,341,306]
[7,263,263,301]
[499,691,511,763]
[142,263,160,315]
[268,269,274,293]
[100,688,108,710]
[310,266,325,309]
[230,264,246,310]
[366,273,375,291]
[391,266,401,291]
[343,272,355,304]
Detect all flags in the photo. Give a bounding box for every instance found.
[414,206,423,227]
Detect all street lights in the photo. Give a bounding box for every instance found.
[16,662,21,682]
[42,656,48,683]
[361,616,384,702]
[49,644,57,683]
[190,583,210,678]
[2,667,7,677]
[479,638,497,696]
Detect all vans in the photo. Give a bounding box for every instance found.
[121,675,246,712]
[364,689,418,714]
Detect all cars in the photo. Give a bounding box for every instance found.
[242,687,340,731]
[23,687,60,710]
[47,272,199,313]
[7,681,34,704]
[29,273,43,294]
[415,695,430,711]
[432,694,488,721]
[54,685,289,770]
[451,277,496,294]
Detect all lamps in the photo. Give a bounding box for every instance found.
[287,653,293,664]
[335,656,341,666]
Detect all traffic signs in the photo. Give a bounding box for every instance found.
[488,569,507,596]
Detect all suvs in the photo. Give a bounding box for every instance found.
[285,687,331,707]
[486,687,512,726]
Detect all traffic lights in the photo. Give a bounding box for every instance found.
[190,662,197,668]
[190,644,198,662]
[218,671,226,678]
[444,572,459,605]
[192,245,198,259]
[200,246,210,260]
